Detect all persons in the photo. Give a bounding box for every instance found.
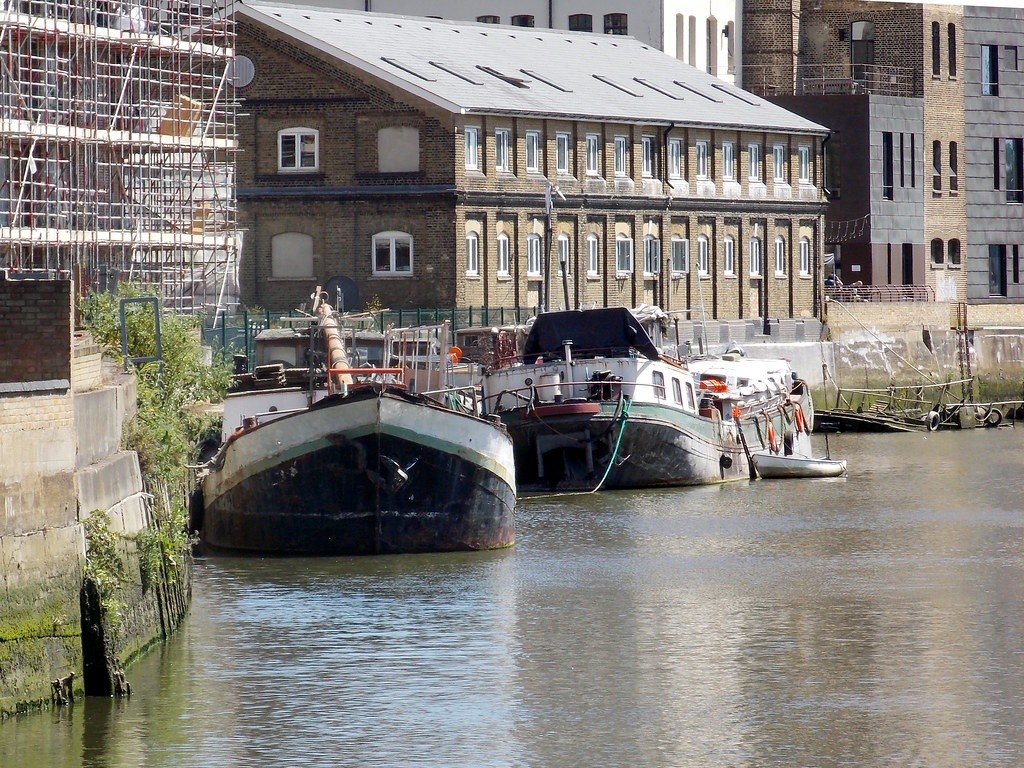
[825,272,868,304]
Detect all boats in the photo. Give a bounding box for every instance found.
[477,304,814,492]
[752,431,849,479]
[184,299,516,557]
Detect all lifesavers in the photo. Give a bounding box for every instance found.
[794,408,803,433]
[769,423,777,451]
